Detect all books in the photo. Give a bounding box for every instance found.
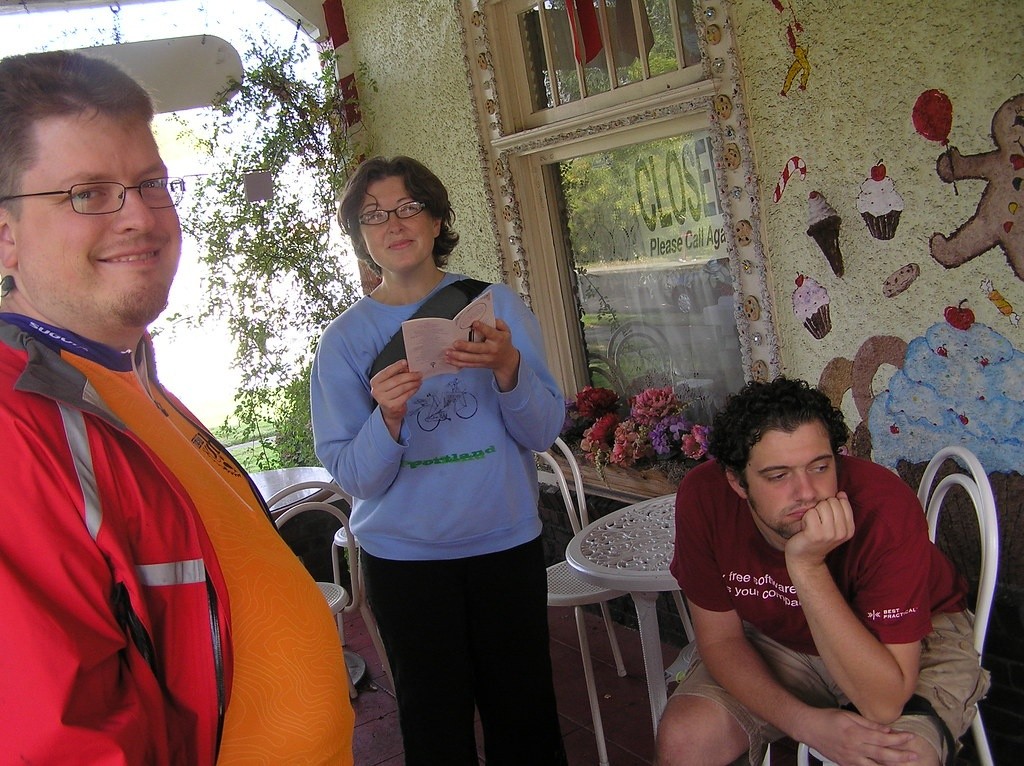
[402,288,496,379]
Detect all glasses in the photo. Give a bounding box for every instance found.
[1,176,186,215]
[358,199,426,225]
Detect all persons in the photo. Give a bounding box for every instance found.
[0,52,355,766]
[655,375,990,766]
[310,156,565,766]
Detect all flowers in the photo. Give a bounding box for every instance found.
[556,380,719,488]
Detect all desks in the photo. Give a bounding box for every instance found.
[246,467,332,516]
[566,492,697,751]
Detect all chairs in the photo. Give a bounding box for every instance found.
[534,436,634,766]
[798,444,998,766]
[265,480,396,708]
